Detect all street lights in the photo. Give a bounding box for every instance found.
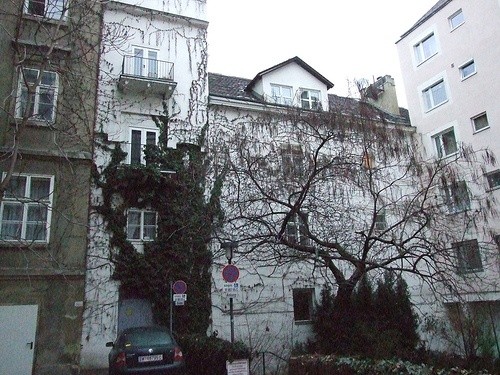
[219,240,236,344]
